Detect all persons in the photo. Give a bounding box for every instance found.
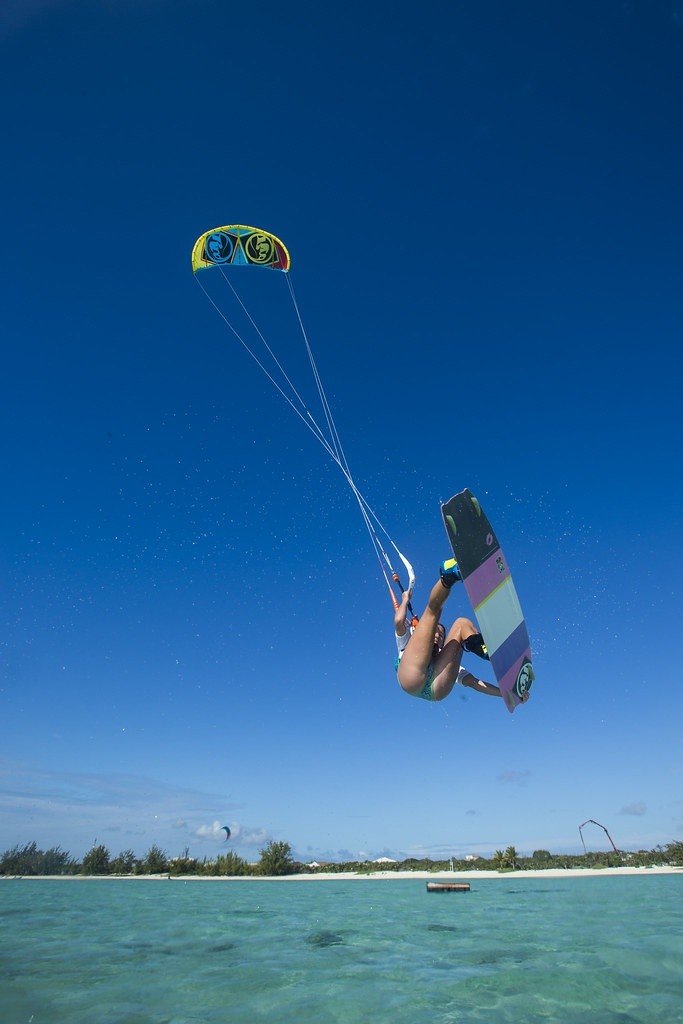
[394,558,530,703]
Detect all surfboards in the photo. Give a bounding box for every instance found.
[434,476,541,722]
